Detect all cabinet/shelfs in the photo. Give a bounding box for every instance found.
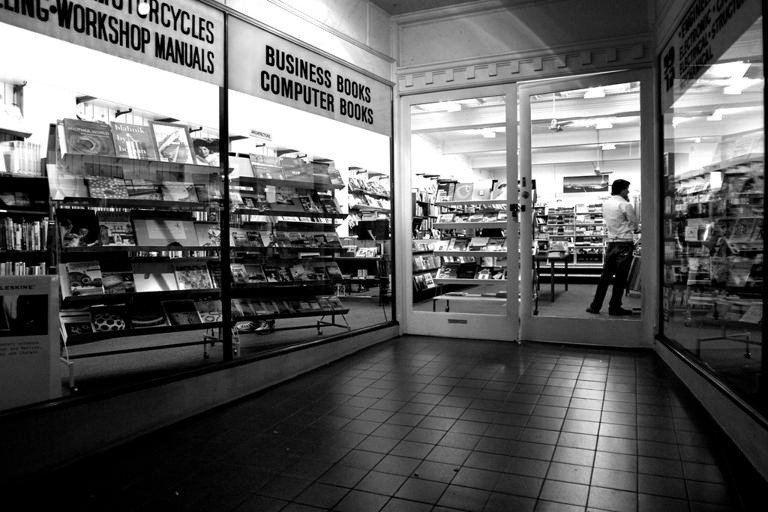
[0,77,394,395]
[662,130,767,394]
[410,171,642,316]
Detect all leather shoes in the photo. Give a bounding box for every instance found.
[586,307,600,314]
[608,305,633,316]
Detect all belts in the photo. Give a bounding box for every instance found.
[608,241,634,245]
